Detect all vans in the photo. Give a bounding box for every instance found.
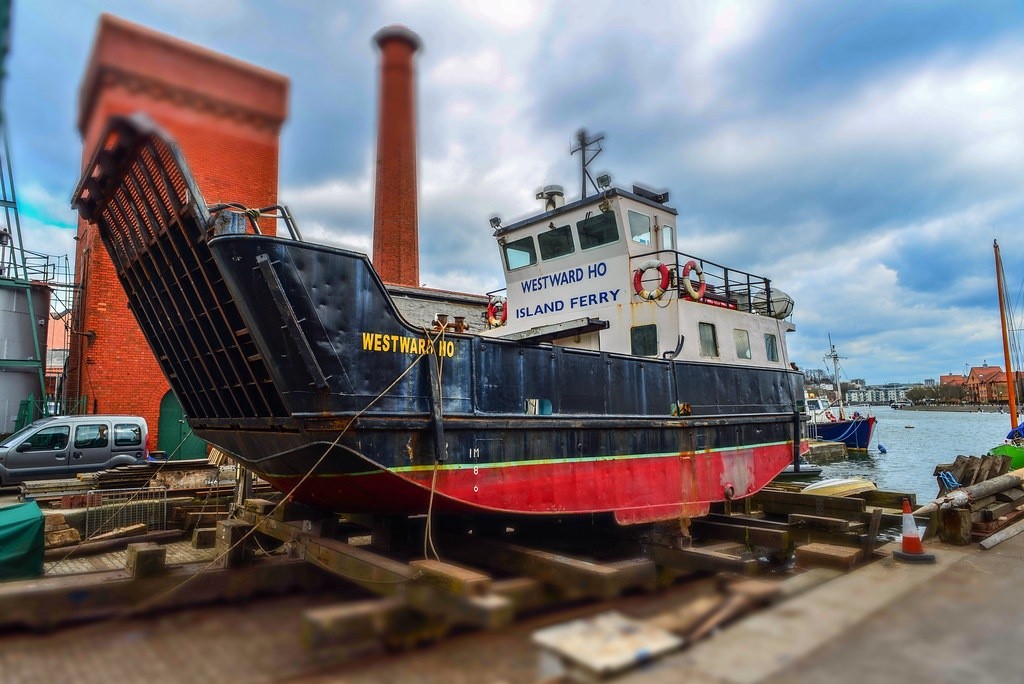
[0,415,149,488]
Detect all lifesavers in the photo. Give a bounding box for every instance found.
[681,260,707,300]
[486,295,507,327]
[825,411,836,420]
[632,257,670,301]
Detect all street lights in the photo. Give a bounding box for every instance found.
[967,391,969,401]
[1000,392,1002,406]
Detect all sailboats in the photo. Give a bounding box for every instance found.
[988,238,1024,472]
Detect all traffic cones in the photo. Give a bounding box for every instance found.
[893,498,936,564]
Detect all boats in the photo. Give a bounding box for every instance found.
[70,112,814,526]
[778,455,822,477]
[805,332,878,451]
[859,404,871,408]
